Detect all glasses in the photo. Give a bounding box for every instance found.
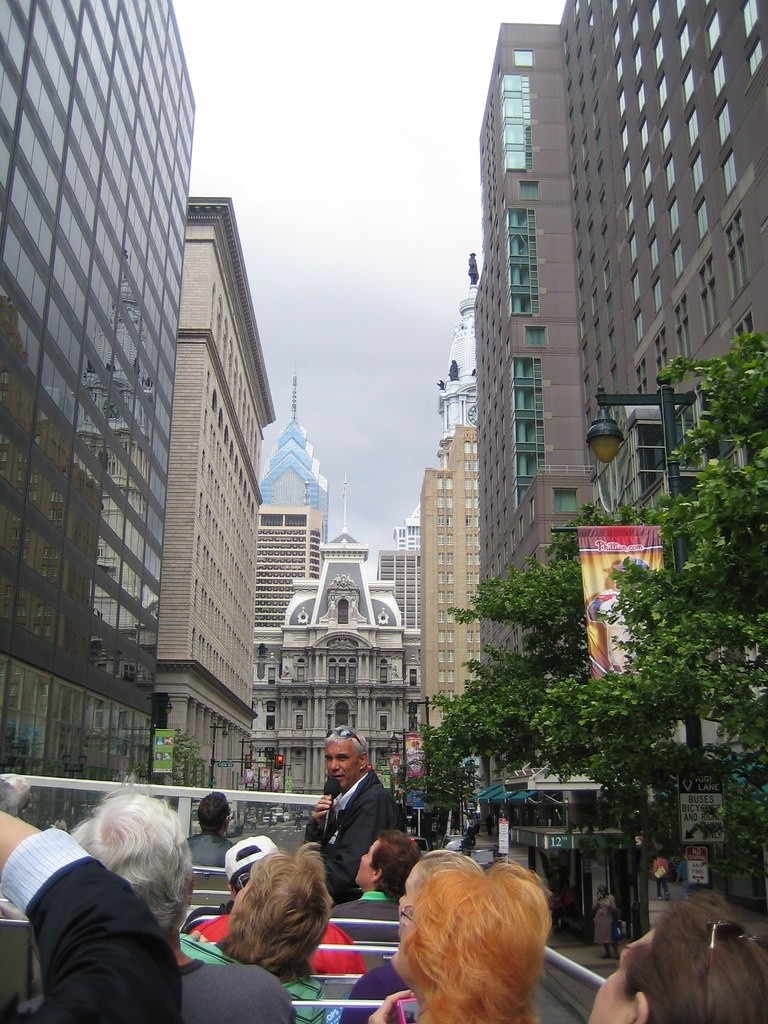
[400,906,417,925]
[326,728,362,746]
[705,919,768,975]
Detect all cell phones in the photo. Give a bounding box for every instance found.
[397,997,420,1024]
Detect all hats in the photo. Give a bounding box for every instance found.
[225,835,279,881]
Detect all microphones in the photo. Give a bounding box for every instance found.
[321,777,341,843]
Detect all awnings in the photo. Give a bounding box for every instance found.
[468,784,536,804]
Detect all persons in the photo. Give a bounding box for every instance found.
[186,791,236,870]
[0,810,182,1024]
[410,812,493,835]
[587,888,768,1024]
[676,855,690,893]
[652,853,670,899]
[592,885,620,960]
[551,883,583,933]
[179,835,551,1024]
[244,808,304,831]
[18,789,295,1024]
[302,725,408,906]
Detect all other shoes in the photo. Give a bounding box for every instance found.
[615,953,619,959]
[658,896,662,900]
[603,954,611,958]
[665,892,669,899]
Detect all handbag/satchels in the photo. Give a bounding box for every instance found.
[611,917,621,939]
[654,858,666,879]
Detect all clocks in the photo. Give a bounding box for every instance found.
[467,404,476,426]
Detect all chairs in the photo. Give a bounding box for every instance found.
[0,865,400,1024]
